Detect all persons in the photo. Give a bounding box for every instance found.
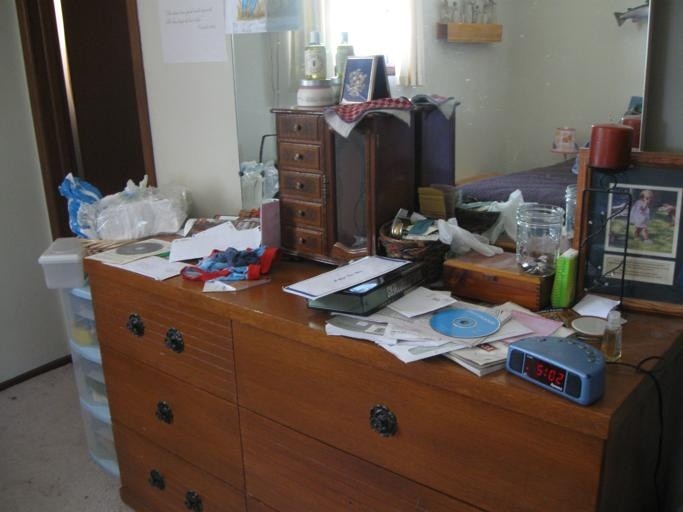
[628,189,657,246]
[657,202,676,216]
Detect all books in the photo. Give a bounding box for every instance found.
[559,249,580,307]
[259,198,281,249]
[549,248,575,309]
[185,216,260,238]
[323,285,581,380]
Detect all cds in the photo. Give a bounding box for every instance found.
[431,306,500,338]
[116,240,162,256]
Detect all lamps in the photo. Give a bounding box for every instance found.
[549,127,579,161]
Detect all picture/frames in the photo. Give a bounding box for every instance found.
[569,146,682,317]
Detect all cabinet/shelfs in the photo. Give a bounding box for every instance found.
[268,97,462,267]
[38,209,683,512]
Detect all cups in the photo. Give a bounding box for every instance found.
[514,203,567,277]
[564,184,577,240]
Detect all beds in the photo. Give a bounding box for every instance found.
[454,157,579,236]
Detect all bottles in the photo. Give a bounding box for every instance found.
[600,310,623,362]
